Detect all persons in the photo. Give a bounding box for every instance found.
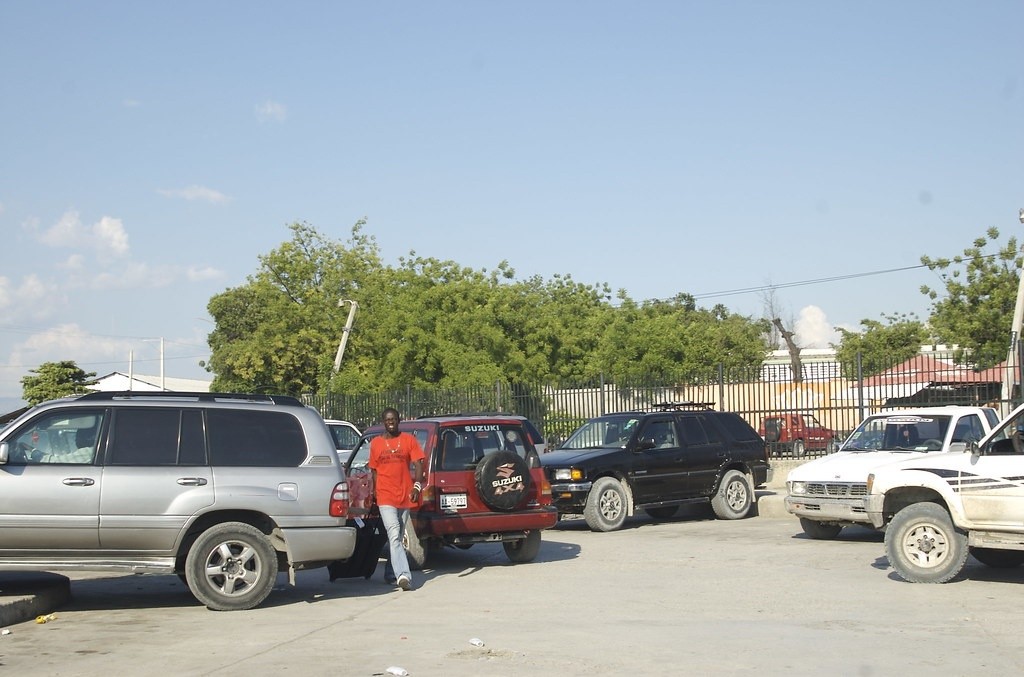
[369,408,426,591]
[20,417,102,463]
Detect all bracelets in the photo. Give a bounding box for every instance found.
[414,481,422,492]
[32,448,36,452]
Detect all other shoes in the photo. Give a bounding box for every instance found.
[384,578,398,585]
[396,574,411,591]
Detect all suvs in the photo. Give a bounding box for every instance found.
[759,414,841,458]
[343,412,558,564]
[540,402,771,532]
[0,389,358,611]
[862,400,1024,584]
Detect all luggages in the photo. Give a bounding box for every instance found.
[326,495,387,582]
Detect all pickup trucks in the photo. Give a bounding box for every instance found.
[784,405,1010,541]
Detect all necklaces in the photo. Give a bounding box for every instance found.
[385,436,400,453]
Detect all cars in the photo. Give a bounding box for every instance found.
[414,413,547,467]
[324,418,366,470]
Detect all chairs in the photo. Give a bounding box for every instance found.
[448,447,474,464]
